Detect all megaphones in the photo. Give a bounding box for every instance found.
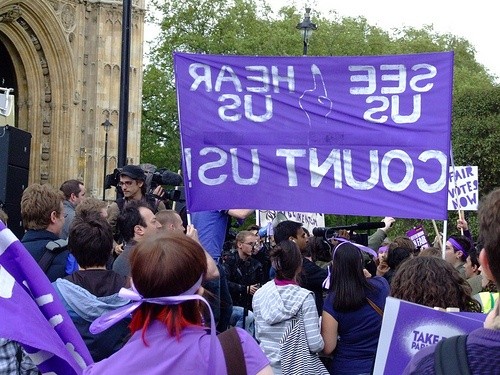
[259,212,288,238]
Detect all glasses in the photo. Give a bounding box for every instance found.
[117,179,136,187]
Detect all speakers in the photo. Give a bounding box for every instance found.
[0,125,31,241]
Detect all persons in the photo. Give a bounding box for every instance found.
[0,164,500,375]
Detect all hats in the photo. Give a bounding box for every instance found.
[117,164,146,180]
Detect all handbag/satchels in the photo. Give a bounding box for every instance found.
[280,318,330,375]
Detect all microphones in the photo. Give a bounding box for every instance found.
[335,222,385,230]
[162,171,182,185]
